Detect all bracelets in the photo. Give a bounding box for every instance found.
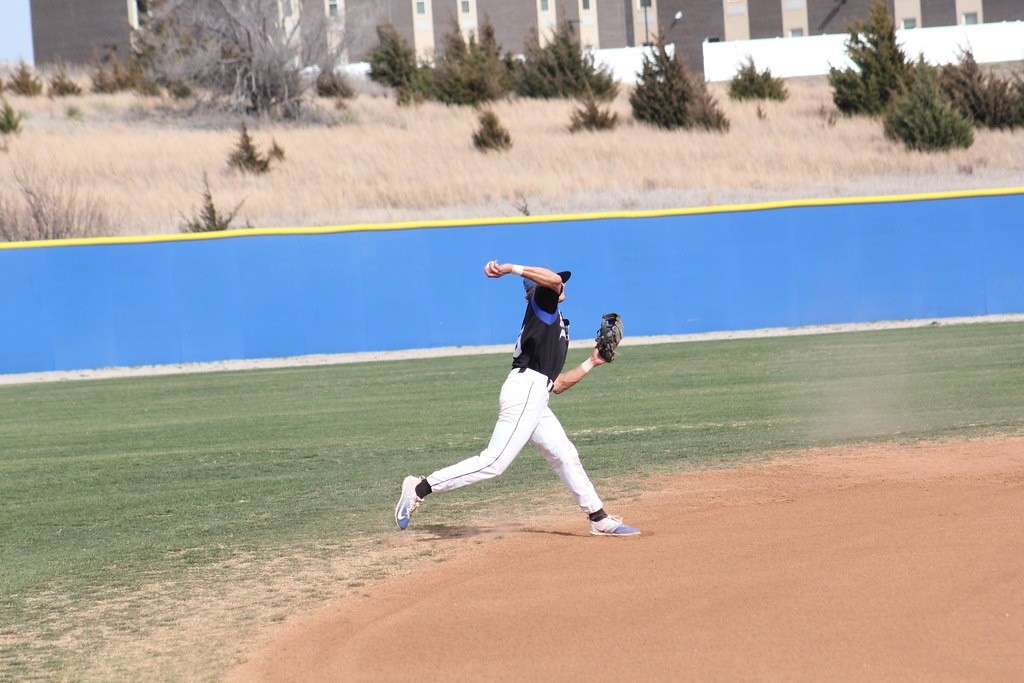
[510,264,524,276]
[581,357,594,373]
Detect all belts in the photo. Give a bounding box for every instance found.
[519,368,555,393]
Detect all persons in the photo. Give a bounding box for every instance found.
[395,259,642,536]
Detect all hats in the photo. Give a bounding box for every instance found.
[523,270,571,293]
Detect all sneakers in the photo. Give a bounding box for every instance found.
[589,515,641,536]
[395,475,424,530]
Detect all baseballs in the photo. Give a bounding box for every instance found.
[486,261,498,273]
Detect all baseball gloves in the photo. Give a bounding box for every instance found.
[594,313,624,364]
